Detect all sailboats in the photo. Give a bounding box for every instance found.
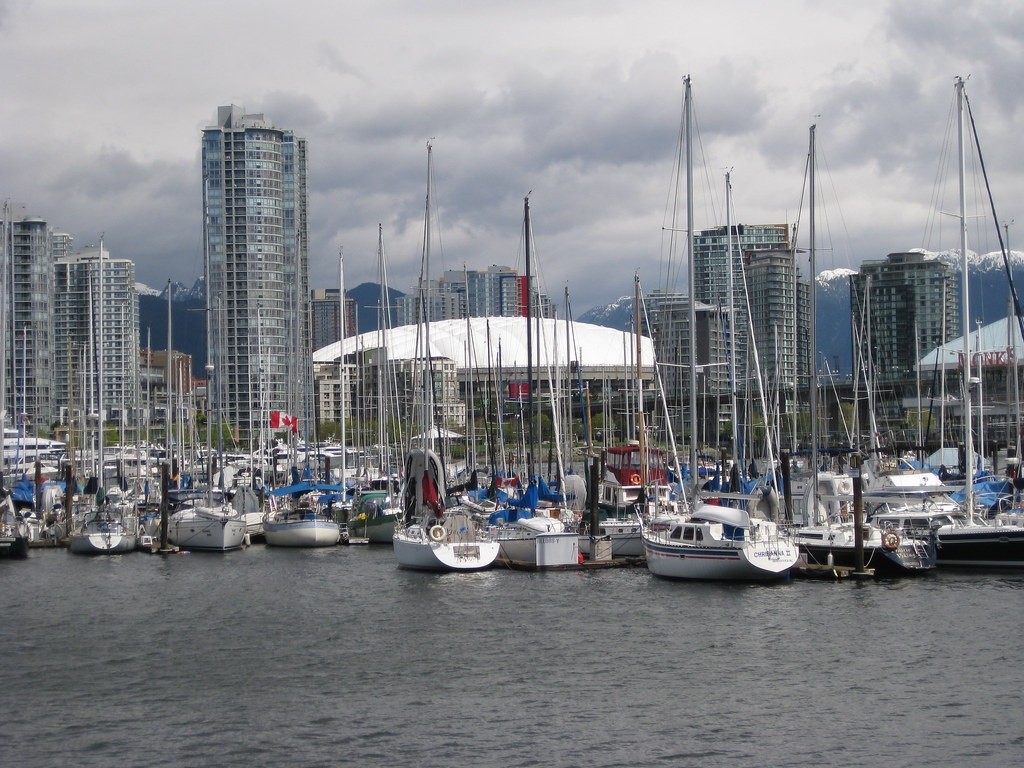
[0,69,1024,582]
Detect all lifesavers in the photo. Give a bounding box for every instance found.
[883,531,901,550]
[630,473,642,485]
[429,525,446,542]
[839,480,853,496]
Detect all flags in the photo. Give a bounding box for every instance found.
[270,411,297,432]
